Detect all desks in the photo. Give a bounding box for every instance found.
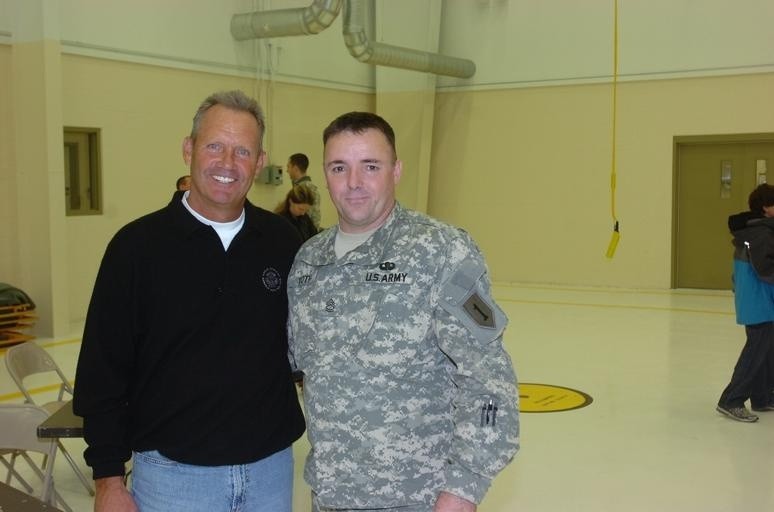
[0,479,64,512]
[36,401,85,503]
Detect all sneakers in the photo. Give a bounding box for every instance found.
[716,401,774,423]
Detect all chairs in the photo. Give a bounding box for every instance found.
[5,342,94,497]
[0,403,58,503]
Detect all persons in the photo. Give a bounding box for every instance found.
[284,108,521,512]
[69,91,307,511]
[168,176,192,203]
[277,184,319,241]
[274,150,322,257]
[715,182,772,424]
